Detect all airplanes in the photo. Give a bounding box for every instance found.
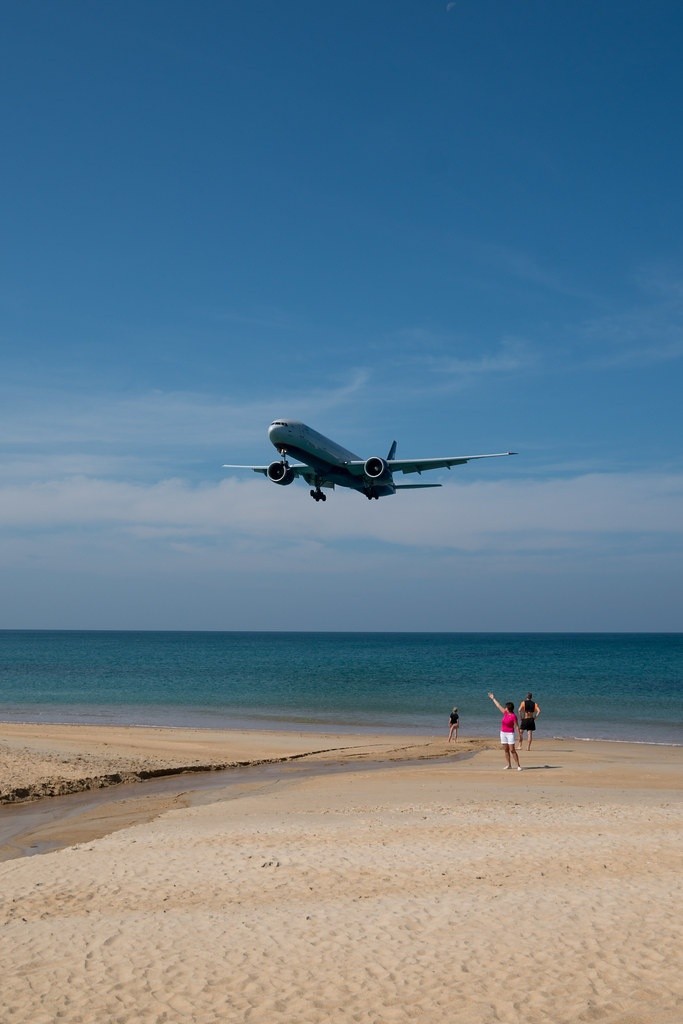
[222,419,519,502]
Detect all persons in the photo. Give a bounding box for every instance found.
[488,692,522,771]
[515,692,541,750]
[447,707,459,742]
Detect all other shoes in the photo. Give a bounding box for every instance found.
[504,766,512,769]
[518,767,521,770]
